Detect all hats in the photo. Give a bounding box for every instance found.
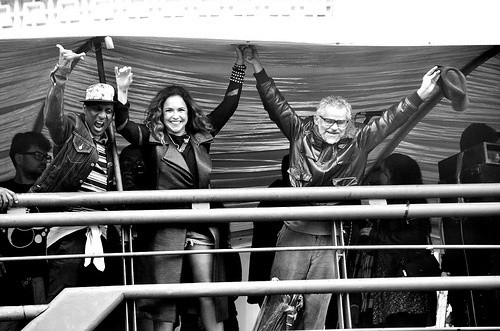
[79,83,117,102]
[431,65,469,111]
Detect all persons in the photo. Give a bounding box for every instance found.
[0,132,53,331]
[29,43,119,305]
[240,44,441,330]
[248,153,441,328]
[114,43,248,331]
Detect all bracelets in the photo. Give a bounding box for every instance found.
[229,64,246,83]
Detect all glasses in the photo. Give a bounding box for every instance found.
[20,151,52,163]
[319,115,349,127]
